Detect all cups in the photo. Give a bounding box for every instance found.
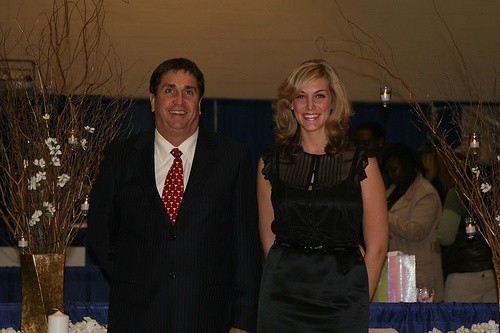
[417,288,435,302]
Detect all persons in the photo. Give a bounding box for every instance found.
[434,153,499,304]
[257,59,390,333]
[84,57,261,333]
[358,121,457,304]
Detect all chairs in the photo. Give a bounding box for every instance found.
[444,270,498,303]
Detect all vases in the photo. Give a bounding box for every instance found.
[19,254,65,333]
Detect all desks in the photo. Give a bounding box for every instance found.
[0,302,109,331]
[368,301,500,333]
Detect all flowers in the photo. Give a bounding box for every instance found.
[448,320,500,333]
[0,0,151,254]
[312,0,500,309]
[68,317,108,333]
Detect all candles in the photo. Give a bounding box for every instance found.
[466,223,475,233]
[471,138,479,148]
[68,134,77,144]
[46,307,70,333]
[18,237,28,247]
[381,87,390,101]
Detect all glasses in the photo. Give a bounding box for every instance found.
[156,87,197,100]
[357,138,372,146]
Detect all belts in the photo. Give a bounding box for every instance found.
[275,240,359,251]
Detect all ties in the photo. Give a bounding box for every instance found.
[161,149,186,226]
